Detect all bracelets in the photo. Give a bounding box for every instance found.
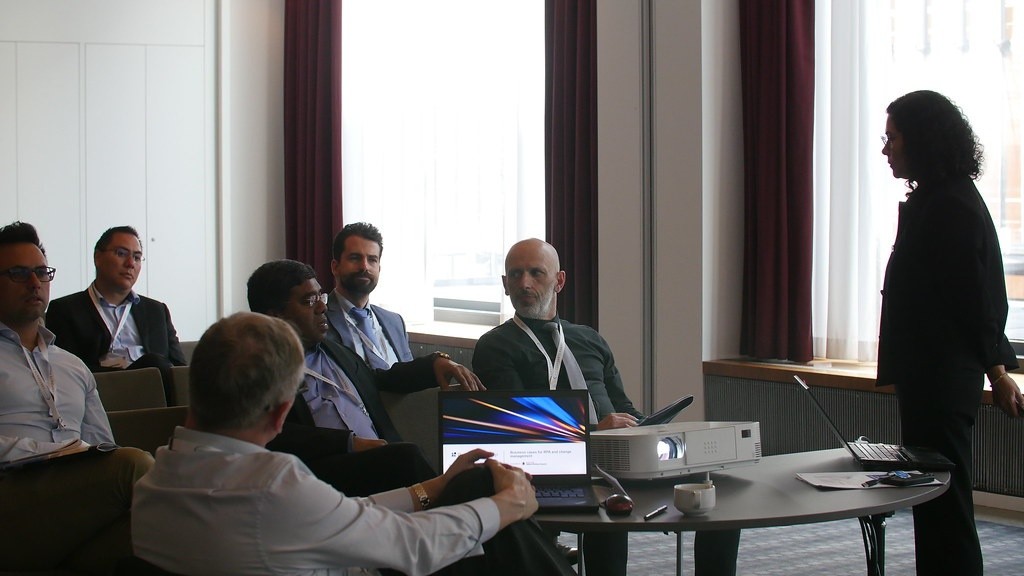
[413,483,430,511]
[990,372,1007,387]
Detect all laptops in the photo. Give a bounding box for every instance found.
[794,374,955,470]
[437,389,600,513]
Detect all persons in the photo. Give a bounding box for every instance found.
[876,90,1024,576]
[0,222,157,576]
[46,226,188,373]
[472,239,741,576]
[131,313,579,576]
[246,261,486,499]
[323,222,579,566]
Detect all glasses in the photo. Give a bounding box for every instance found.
[105,248,143,263]
[285,293,329,308]
[264,380,309,412]
[0,266,56,283]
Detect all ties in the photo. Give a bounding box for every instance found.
[544,319,597,429]
[351,307,390,372]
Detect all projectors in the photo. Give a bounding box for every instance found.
[589,421,763,480]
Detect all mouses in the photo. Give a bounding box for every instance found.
[606,494,638,516]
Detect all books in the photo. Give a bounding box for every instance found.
[0,438,121,471]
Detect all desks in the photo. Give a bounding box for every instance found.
[533,447,951,576]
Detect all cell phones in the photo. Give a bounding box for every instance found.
[879,474,935,486]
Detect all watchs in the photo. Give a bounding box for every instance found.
[435,351,452,360]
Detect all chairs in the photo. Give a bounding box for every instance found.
[94,367,465,478]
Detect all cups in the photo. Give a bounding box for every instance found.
[674,483,716,517]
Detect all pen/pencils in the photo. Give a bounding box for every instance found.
[644,505,667,520]
